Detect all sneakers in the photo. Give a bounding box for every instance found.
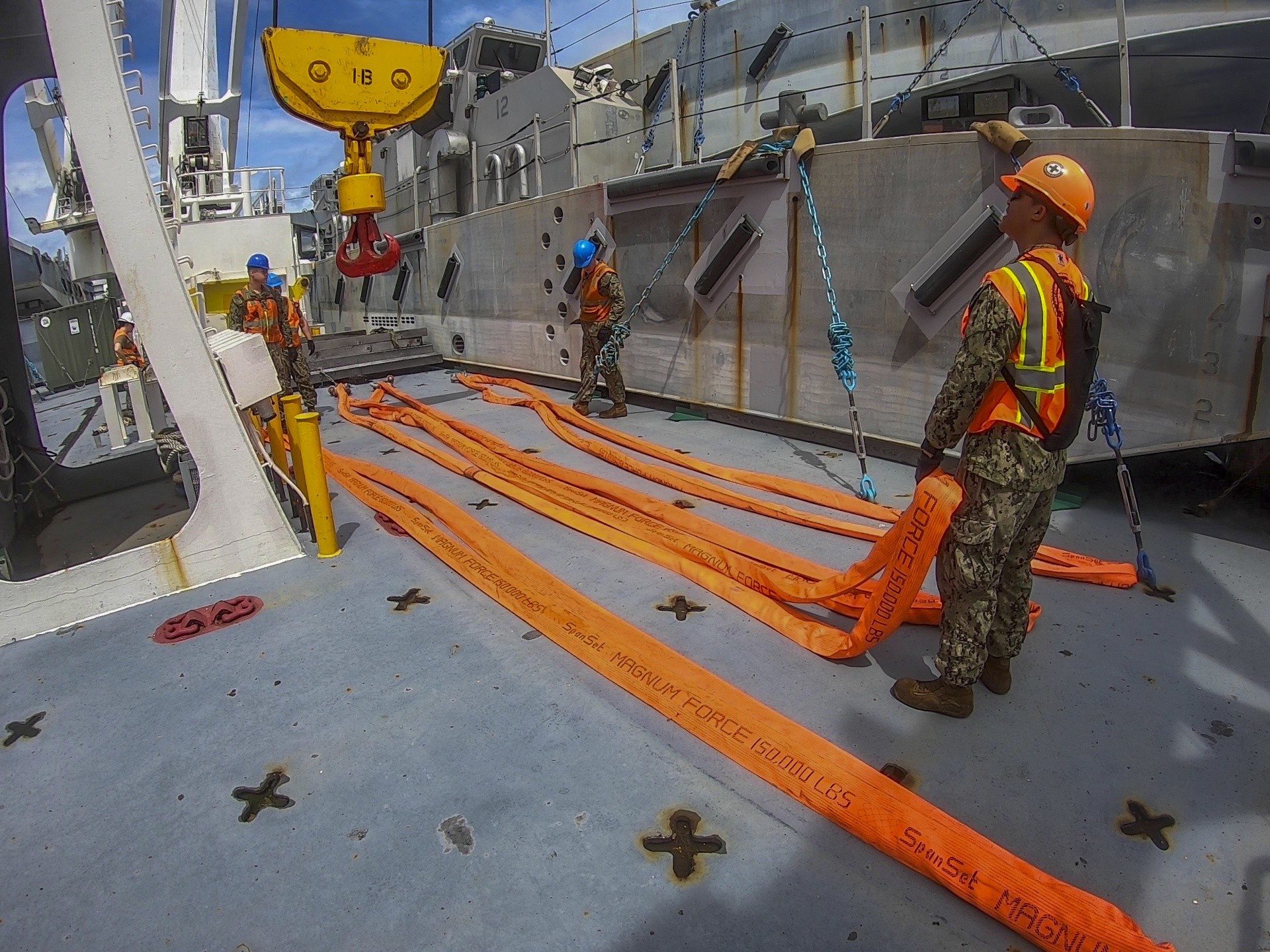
[980,655,1011,694]
[572,403,589,415]
[893,676,974,718]
[599,402,627,418]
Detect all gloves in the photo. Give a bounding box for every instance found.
[597,325,612,343]
[288,346,298,363]
[915,451,942,485]
[308,340,315,356]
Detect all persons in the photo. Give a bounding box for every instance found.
[113,313,145,425]
[891,155,1097,718]
[228,253,321,434]
[571,240,628,418]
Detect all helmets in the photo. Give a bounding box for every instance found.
[246,254,272,270]
[117,312,134,324]
[1000,154,1094,232]
[573,240,596,268]
[266,273,283,287]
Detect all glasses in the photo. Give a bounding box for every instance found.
[1010,189,1047,206]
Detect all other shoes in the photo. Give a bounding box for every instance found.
[309,409,320,421]
